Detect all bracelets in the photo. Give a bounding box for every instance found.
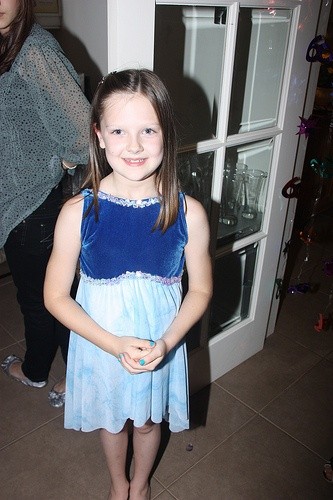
[62,160,77,170]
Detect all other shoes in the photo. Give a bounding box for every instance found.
[48,382,66,408]
[1,354,46,388]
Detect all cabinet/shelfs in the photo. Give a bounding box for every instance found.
[61,0,333,394]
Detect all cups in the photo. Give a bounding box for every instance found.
[177,159,264,225]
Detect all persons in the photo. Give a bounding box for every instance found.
[0,1,106,411]
[39,68,215,500]
